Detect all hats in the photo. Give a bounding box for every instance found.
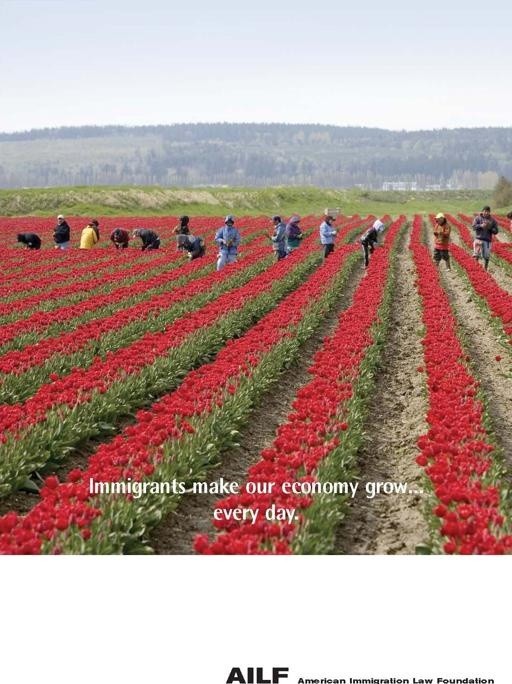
[225,215,235,224]
[374,220,384,231]
[58,215,63,219]
[435,213,444,218]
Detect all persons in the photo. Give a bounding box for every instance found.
[15,204,511,272]
